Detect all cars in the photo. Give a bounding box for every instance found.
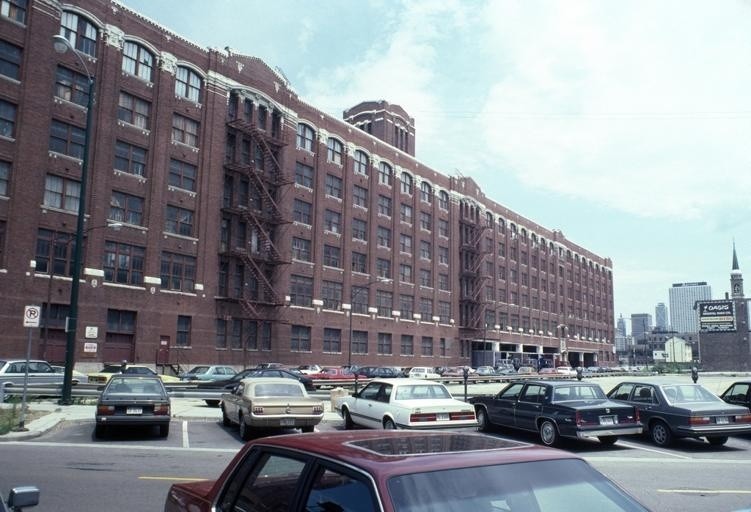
[0,484,41,511]
[468,377,645,446]
[221,377,327,441]
[197,367,318,406]
[1,357,90,400]
[85,360,181,384]
[719,382,751,408]
[164,428,652,512]
[604,380,751,448]
[94,371,173,437]
[333,377,481,435]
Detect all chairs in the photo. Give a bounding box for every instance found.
[116,384,128,392]
[11,365,16,373]
[21,366,25,373]
[666,388,676,398]
[640,389,651,397]
[144,385,155,392]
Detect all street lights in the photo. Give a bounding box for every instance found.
[349,278,394,365]
[43,221,124,361]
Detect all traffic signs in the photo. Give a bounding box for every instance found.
[23,305,41,329]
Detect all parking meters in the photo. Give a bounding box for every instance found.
[577,366,585,396]
[119,359,127,384]
[692,366,699,385]
[350,367,360,395]
[459,365,468,401]
